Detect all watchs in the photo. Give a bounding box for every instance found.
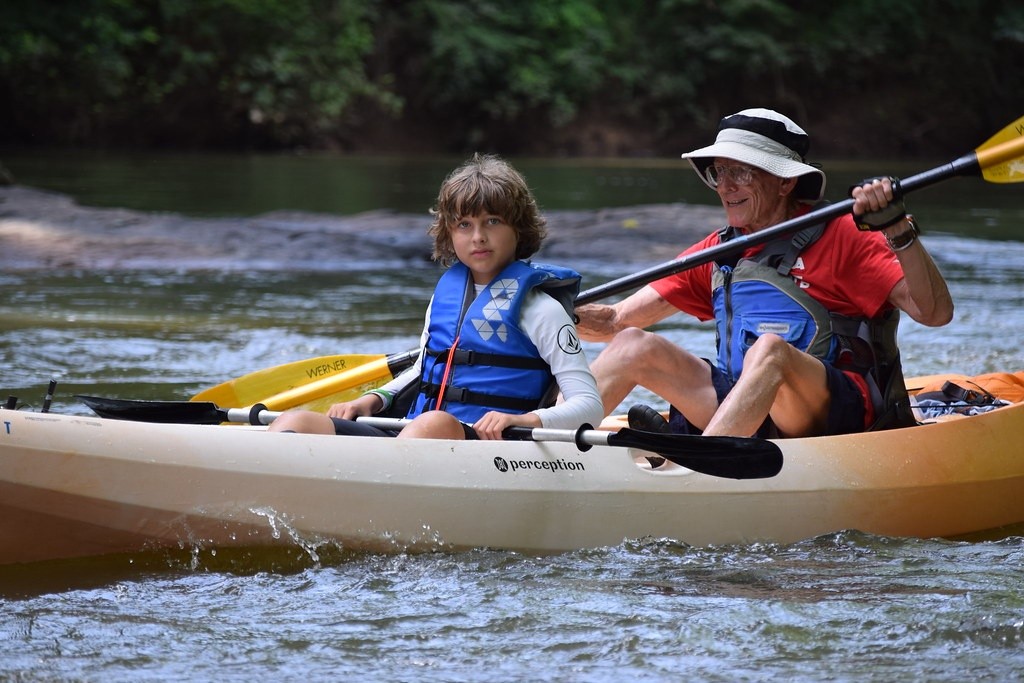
[882,215,920,252]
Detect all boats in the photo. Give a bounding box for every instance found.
[0,371,1024,564]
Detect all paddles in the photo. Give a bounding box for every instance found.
[79,392,785,480]
[188,117,1024,428]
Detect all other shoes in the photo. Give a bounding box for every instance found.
[627,404,671,434]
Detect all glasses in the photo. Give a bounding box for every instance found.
[705,164,765,186]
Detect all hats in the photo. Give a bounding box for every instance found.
[681,108,827,204]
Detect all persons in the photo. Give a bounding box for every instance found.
[266,154,605,440]
[552,106,955,438]
